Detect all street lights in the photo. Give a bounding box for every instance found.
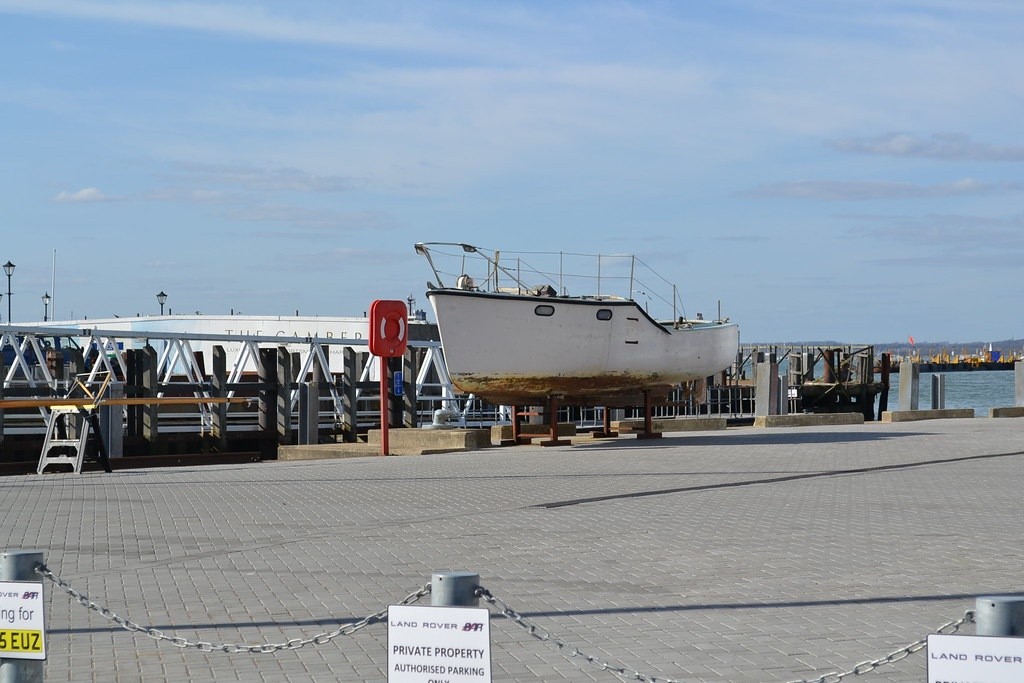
[41,292,52,322]
[156,292,168,317]
[3,260,16,324]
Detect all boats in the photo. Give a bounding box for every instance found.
[413,238,740,407]
